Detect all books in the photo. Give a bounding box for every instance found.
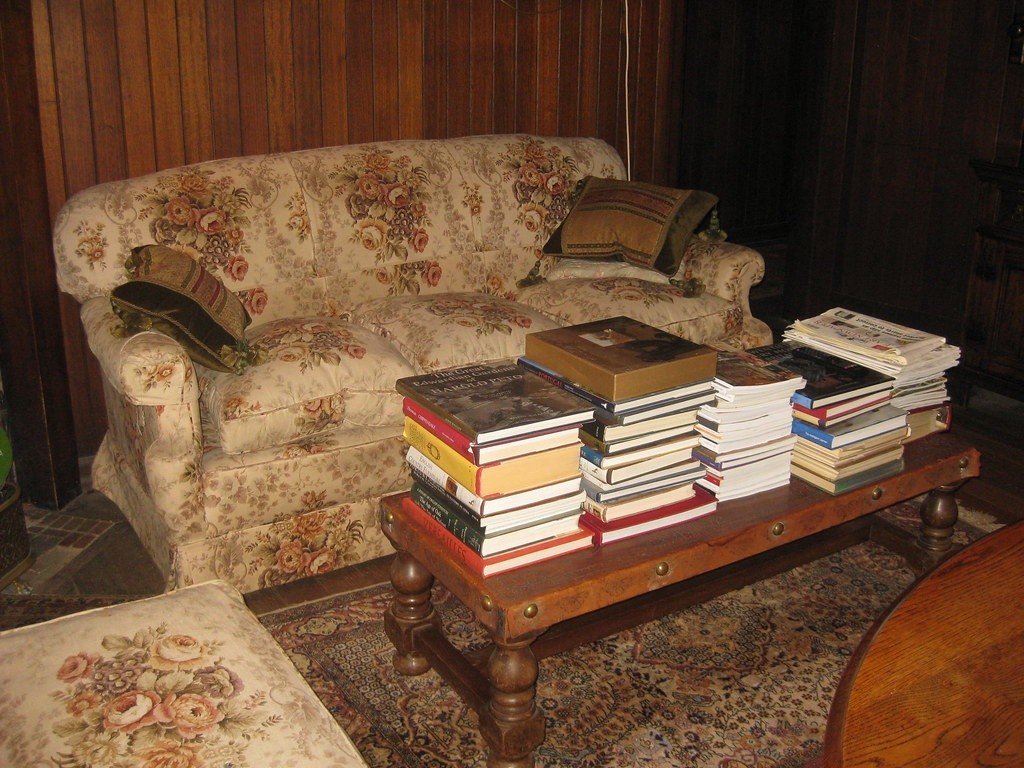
[394,307,962,580]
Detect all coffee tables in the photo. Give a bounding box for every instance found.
[378,388,983,767]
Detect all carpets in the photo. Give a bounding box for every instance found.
[240,488,991,767]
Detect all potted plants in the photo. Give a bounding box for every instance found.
[0,429,35,592]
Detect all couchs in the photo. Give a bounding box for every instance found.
[0,578,370,768]
[51,133,773,597]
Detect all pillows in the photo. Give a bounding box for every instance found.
[109,243,256,375]
[515,174,728,300]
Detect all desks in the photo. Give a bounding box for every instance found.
[821,518,1024,768]
